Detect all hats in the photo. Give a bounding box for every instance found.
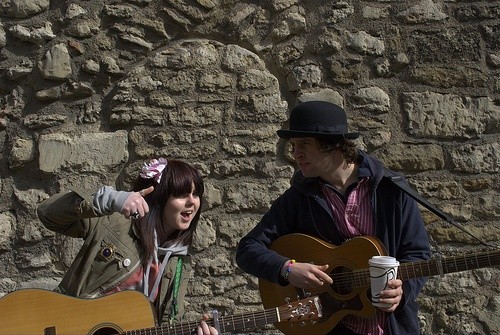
[276,100,359,140]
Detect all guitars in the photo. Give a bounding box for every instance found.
[0,288,323,334]
[258,232,500,335]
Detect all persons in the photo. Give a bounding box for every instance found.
[36,159,221,334]
[236,101,423,335]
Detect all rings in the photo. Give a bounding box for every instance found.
[130,211,140,220]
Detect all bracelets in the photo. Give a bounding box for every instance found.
[284,258,296,280]
